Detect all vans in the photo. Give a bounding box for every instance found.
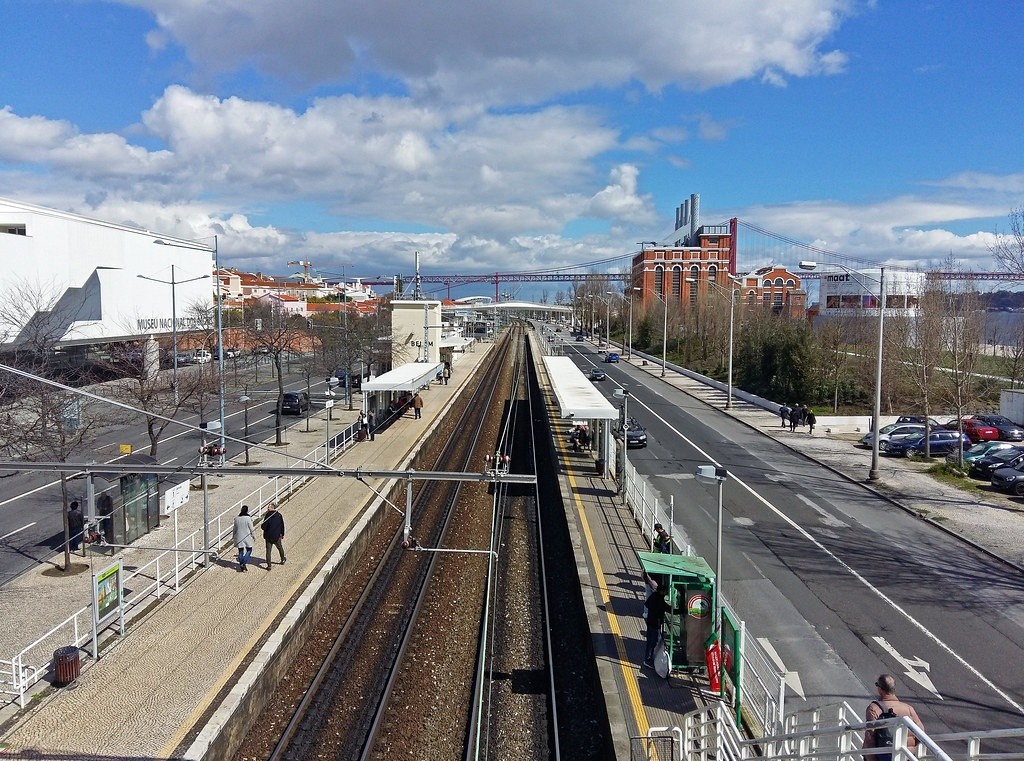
[863,423,935,450]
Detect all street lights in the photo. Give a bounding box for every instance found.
[633,286,668,378]
[694,464,728,664]
[684,275,740,411]
[137,263,210,407]
[799,260,889,485]
[557,295,612,349]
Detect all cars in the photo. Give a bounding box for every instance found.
[970,414,1024,442]
[605,291,637,361]
[970,445,1024,480]
[940,418,999,442]
[945,441,1016,466]
[575,335,585,342]
[614,418,649,449]
[555,328,562,332]
[335,369,362,388]
[98,347,268,365]
[588,368,608,381]
[990,460,1023,495]
[885,431,972,459]
[612,388,629,506]
[605,353,620,363]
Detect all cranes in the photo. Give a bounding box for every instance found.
[286,255,357,274]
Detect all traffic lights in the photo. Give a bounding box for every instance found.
[306,320,314,330]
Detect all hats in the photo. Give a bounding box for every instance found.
[654,524,662,531]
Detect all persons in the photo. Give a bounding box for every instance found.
[653,523,670,554]
[861,675,924,761]
[565,424,590,452]
[233,506,255,572]
[439,367,449,385]
[779,403,788,428]
[358,409,375,442]
[69,502,83,551]
[388,390,412,420]
[806,411,815,434]
[97,492,110,525]
[411,394,424,419]
[641,573,658,637]
[261,504,286,570]
[787,403,808,432]
[644,586,687,670]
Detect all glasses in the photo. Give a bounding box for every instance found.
[875,682,885,690]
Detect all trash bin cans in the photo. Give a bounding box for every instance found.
[53,645,80,683]
[358,429,366,441]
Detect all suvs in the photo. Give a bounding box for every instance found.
[896,415,948,431]
[277,391,312,415]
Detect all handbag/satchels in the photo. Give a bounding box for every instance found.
[872,700,899,761]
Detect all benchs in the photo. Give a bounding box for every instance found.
[576,438,591,453]
[662,624,685,676]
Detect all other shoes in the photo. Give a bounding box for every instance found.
[240,563,248,571]
[266,567,271,570]
[281,557,286,564]
[644,658,655,669]
